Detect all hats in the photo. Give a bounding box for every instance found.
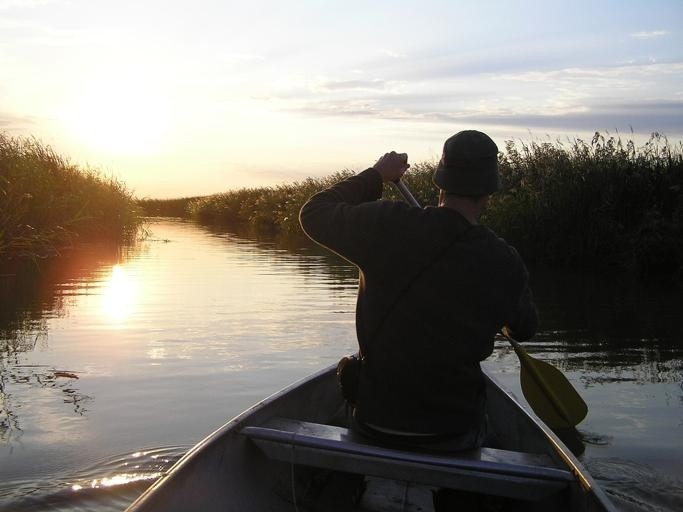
[432,130,499,196]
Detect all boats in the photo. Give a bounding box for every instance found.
[123,349,615,509]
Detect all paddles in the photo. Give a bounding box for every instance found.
[395,179,588,428]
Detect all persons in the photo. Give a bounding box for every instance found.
[299,129,541,451]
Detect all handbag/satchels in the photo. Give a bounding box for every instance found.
[336,354,369,400]
